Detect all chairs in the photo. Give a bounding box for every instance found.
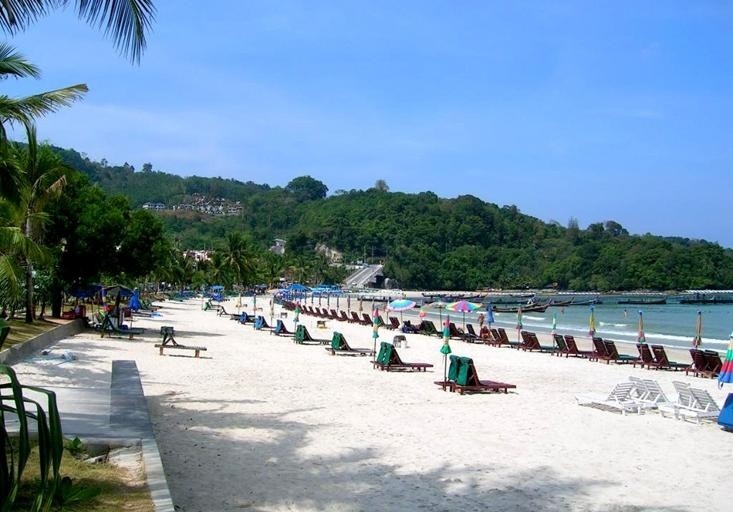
[99,310,144,339]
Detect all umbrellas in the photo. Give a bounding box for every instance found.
[693,310,701,376]
[202,286,494,333]
[718,332,733,384]
[68,281,141,325]
[515,304,523,349]
[373,308,379,370]
[440,316,451,392]
[588,306,597,360]
[550,312,558,353]
[637,310,646,362]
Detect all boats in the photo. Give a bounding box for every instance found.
[618,298,667,304]
[358,295,394,302]
[420,292,602,312]
[680,297,733,303]
